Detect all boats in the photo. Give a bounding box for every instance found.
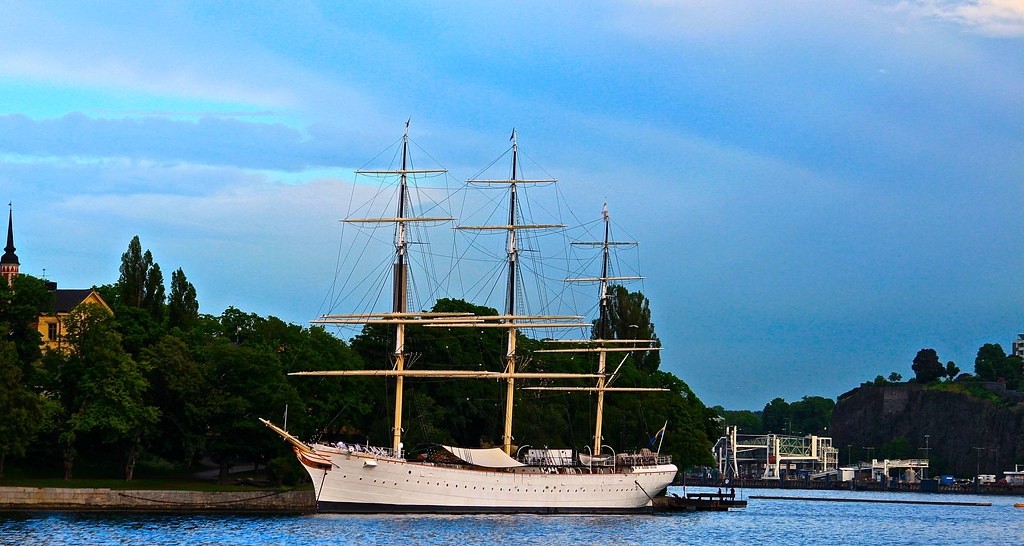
[647,465,749,512]
[1004,464,1024,485]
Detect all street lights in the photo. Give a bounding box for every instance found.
[847,444,852,464]
[863,446,874,464]
[784,418,791,454]
[973,446,985,474]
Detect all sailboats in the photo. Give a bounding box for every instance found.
[256,112,679,514]
